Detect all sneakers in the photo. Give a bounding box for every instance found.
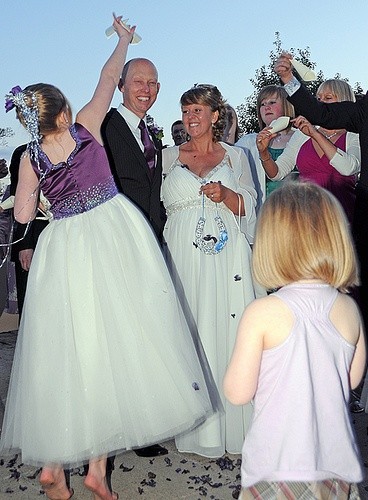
[349,402,364,412]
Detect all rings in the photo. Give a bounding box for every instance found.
[211,194,214,198]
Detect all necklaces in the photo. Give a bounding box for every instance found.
[319,128,341,141]
[275,134,285,143]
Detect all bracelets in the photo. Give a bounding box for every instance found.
[258,152,272,161]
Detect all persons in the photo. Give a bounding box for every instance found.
[223,104,239,146]
[101,57,169,458]
[256,80,365,213]
[0,12,215,500]
[222,180,366,500]
[0,159,9,318]
[222,85,299,217]
[171,120,188,147]
[9,142,49,327]
[160,84,257,459]
[274,51,368,412]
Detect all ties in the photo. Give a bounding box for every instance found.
[138,119,155,176]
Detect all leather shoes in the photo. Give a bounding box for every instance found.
[133,444,169,458]
